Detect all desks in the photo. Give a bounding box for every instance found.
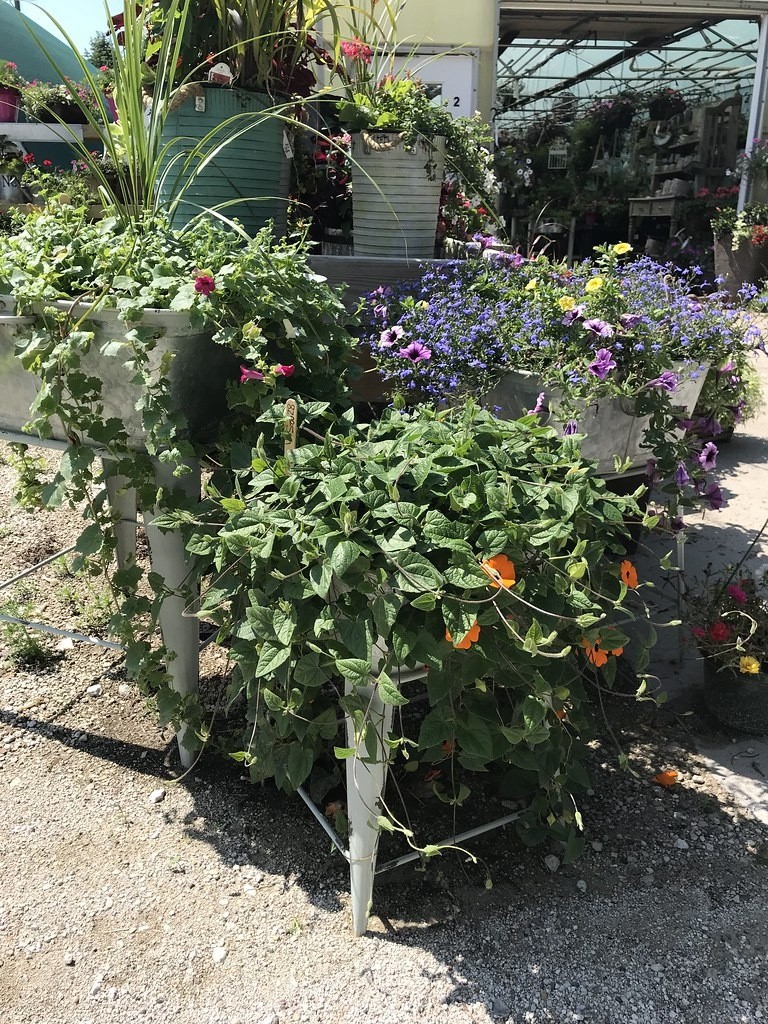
[509,209,580,269]
[0,300,204,769]
[628,195,683,258]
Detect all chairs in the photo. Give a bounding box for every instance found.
[288,571,564,937]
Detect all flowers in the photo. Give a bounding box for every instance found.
[0,0,768,897]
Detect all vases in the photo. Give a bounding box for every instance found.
[40,101,89,125]
[608,110,631,128]
[351,129,445,259]
[713,232,765,302]
[483,358,709,474]
[0,85,22,123]
[586,213,597,226]
[697,644,768,736]
[153,87,287,245]
[648,97,685,120]
[271,134,291,249]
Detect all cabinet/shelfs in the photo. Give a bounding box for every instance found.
[630,92,742,197]
